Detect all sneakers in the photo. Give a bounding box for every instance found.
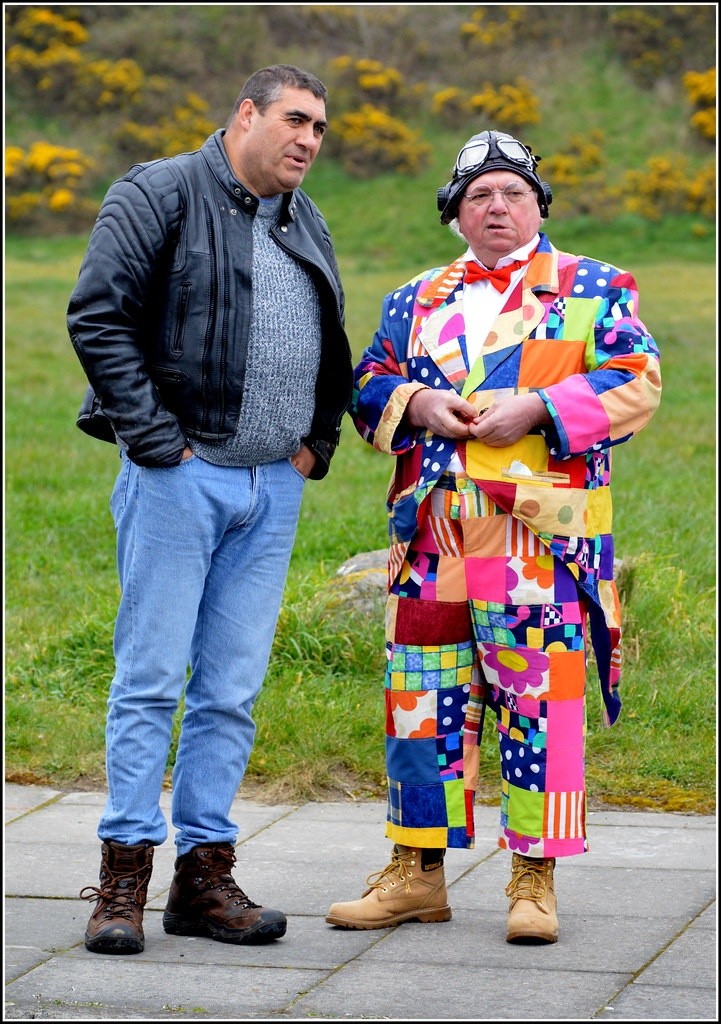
[505,853,558,942]
[80,842,154,952]
[163,844,287,944]
[326,844,452,930]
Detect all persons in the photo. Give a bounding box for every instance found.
[68,63,354,954]
[324,130,661,941]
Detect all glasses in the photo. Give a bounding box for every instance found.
[456,139,531,175]
[463,181,533,208]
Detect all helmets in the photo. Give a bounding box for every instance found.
[437,131,553,226]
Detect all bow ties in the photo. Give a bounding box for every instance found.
[463,262,511,294]
